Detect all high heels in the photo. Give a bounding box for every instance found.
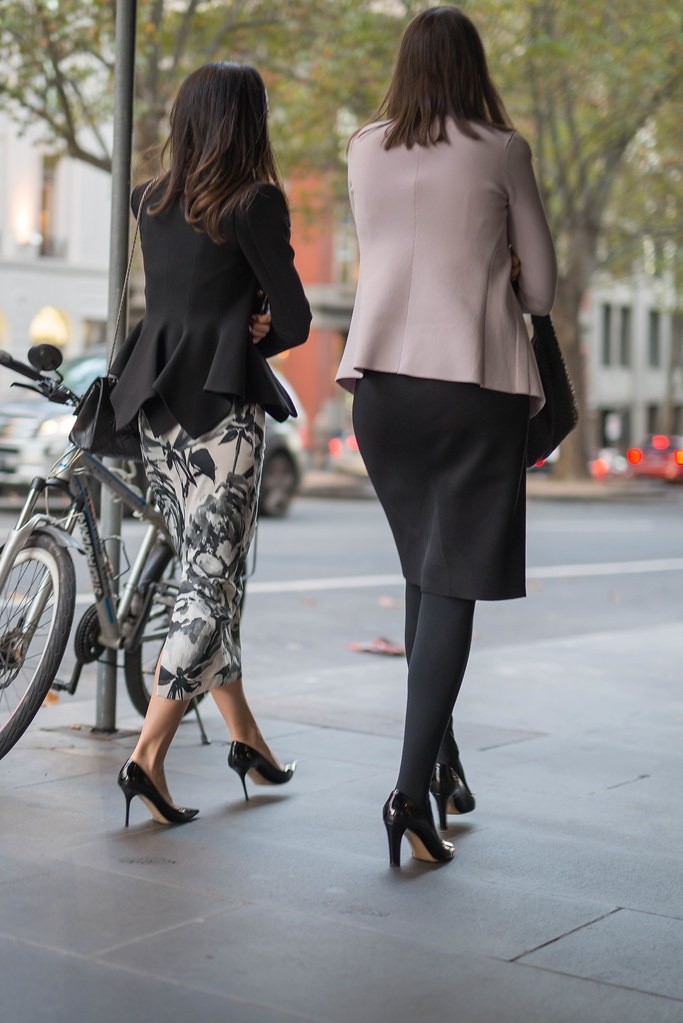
[430,763,476,832]
[382,788,456,868]
[117,758,200,828]
[227,740,298,801]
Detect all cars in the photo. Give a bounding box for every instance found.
[0,352,310,522]
[624,435,682,485]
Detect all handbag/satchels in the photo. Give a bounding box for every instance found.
[69,378,142,457]
[528,314,576,468]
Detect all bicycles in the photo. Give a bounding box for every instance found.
[0,341,217,758]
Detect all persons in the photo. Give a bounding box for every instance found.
[109,61,315,830]
[335,6,557,868]
